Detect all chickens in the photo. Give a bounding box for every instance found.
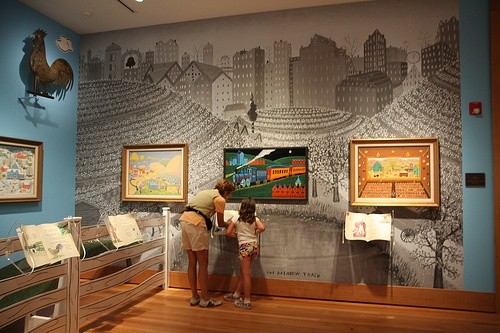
[25,28,74,100]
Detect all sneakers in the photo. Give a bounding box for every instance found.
[198,298,222,307]
[224,294,242,301]
[234,300,252,308]
[189,296,200,305]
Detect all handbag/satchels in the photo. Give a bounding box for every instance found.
[206,218,213,230]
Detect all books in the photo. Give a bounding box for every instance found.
[104,213,144,249]
[344,209,393,243]
[214,206,257,236]
[16,219,80,270]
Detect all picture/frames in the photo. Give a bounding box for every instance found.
[122,143,188,203]
[350,138,440,208]
[0,136,44,204]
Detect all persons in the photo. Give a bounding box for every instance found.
[225,198,265,310]
[177,179,235,308]
